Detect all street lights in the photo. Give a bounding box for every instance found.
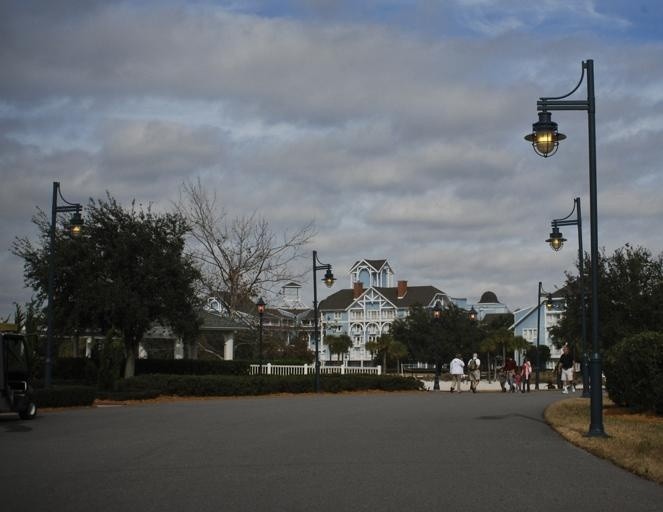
[534,281,553,389]
[255,297,267,375]
[45,181,84,388]
[524,59,613,438]
[312,250,336,392]
[468,305,478,327]
[545,197,592,398]
[432,304,442,391]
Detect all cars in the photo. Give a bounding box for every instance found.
[0,323,39,420]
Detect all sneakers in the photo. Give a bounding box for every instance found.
[451,387,575,394]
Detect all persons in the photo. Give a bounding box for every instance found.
[449,345,577,395]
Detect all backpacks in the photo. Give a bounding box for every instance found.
[470,359,477,371]
